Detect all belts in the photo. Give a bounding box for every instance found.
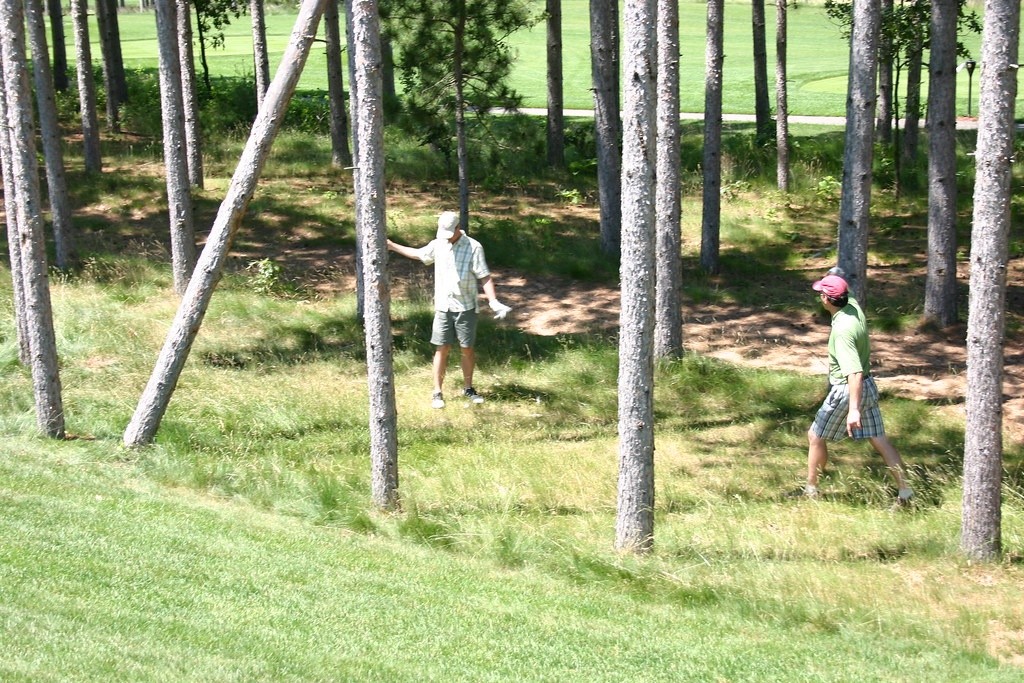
[862,373,873,381]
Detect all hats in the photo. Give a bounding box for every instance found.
[812,275,849,299]
[437,212,460,238]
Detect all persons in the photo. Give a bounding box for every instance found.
[786,268,917,511]
[385,212,511,409]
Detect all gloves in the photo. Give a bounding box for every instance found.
[489,300,512,320]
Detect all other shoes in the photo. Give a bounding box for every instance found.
[788,485,829,501]
[431,392,444,409]
[464,386,484,403]
[890,492,914,514]
